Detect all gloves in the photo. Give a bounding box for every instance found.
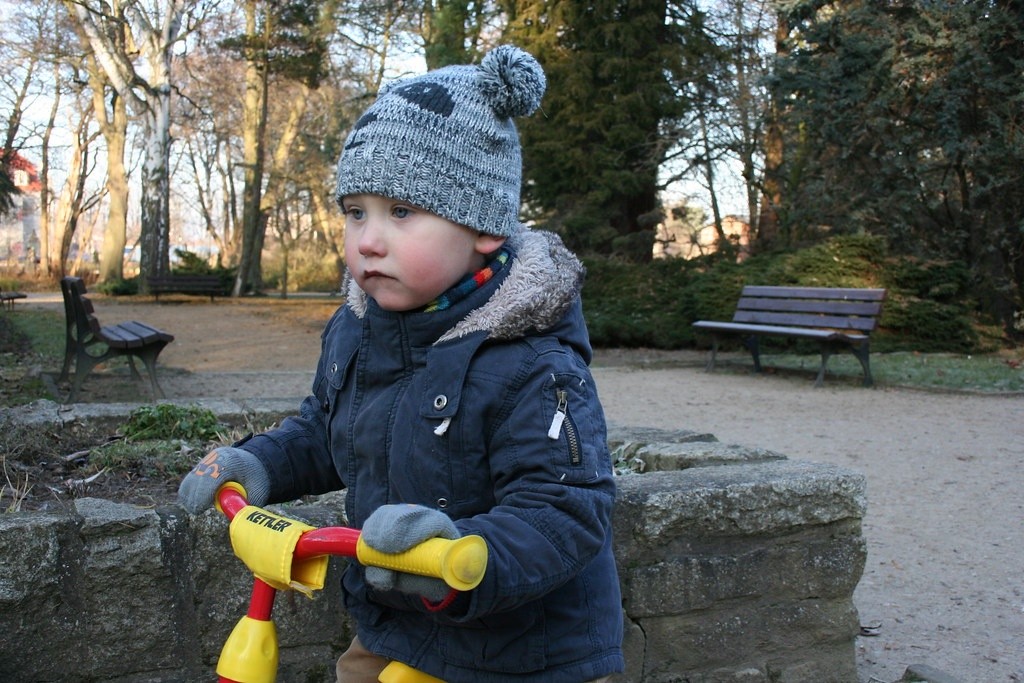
[363,503,462,603]
[177,447,270,517]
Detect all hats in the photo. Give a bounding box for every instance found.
[335,44,548,240]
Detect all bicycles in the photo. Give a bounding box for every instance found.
[212,473,489,683]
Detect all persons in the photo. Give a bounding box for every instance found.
[176,45,626,683]
[27,247,35,266]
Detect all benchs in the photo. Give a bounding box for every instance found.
[0,287,28,314]
[147,276,221,303]
[55,277,175,405]
[693,285,886,390]
